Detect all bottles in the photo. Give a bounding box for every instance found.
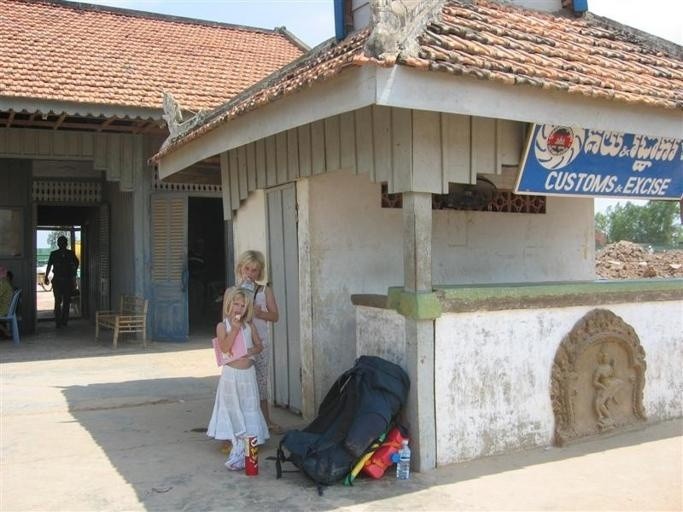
[397,439,411,482]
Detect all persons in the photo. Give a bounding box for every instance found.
[205,286,270,453]
[234,249,279,425]
[45,236,80,329]
[8,271,15,287]
[0,265,14,337]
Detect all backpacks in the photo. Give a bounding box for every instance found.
[276,355,410,486]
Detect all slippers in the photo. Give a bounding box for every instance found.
[268,424,285,434]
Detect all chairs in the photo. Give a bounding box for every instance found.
[1,288,23,345]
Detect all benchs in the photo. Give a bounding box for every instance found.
[94,295,150,349]
